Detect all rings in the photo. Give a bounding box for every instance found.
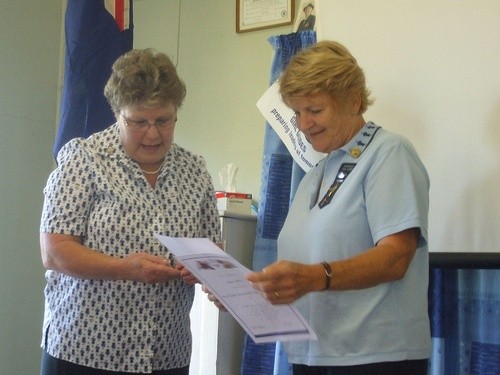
[275,291,280,298]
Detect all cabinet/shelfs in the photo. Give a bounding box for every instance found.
[189,211,260,375]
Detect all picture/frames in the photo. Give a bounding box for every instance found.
[236,0,295,33]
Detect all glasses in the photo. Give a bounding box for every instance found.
[119,112,176,130]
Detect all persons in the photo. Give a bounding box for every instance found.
[39,48,224,375]
[296,1,316,32]
[202,40,432,375]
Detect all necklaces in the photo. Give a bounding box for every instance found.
[142,161,163,175]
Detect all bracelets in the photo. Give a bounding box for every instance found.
[320,261,332,290]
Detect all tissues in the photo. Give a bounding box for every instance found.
[213,161,252,215]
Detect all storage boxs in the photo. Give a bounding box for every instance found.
[215,192,252,212]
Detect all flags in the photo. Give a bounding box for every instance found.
[54,1,134,167]
